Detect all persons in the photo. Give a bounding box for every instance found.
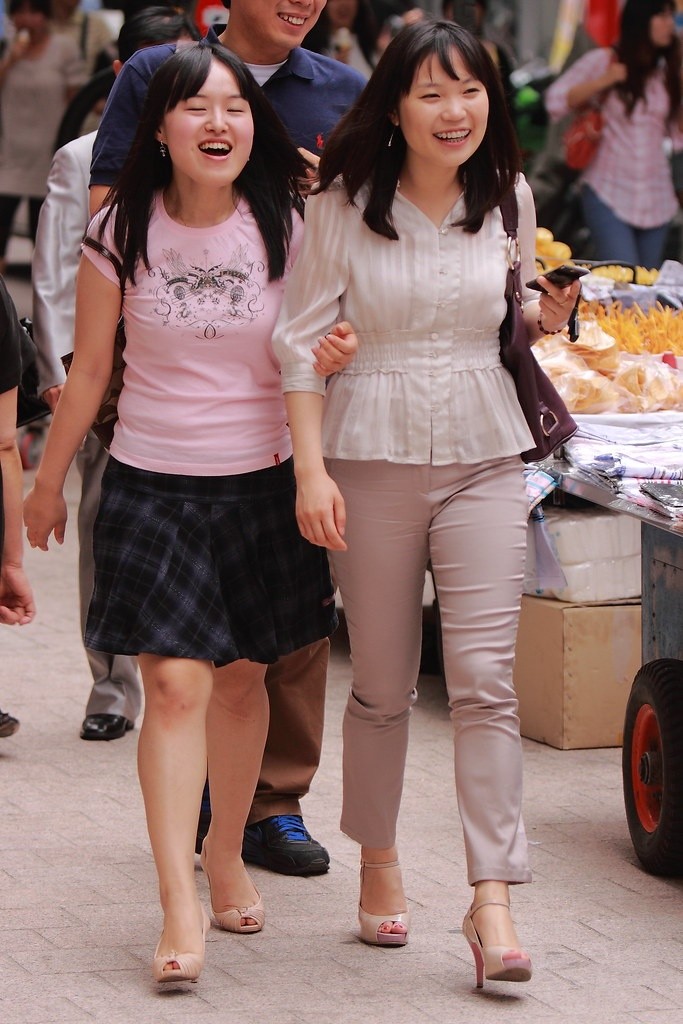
[88,0,370,876]
[546,0,683,267]
[30,5,201,740]
[443,0,516,130]
[0,275,35,739]
[52,0,192,153]
[271,18,580,989]
[23,43,359,983]
[48,0,112,75]
[301,0,375,77]
[0,0,73,276]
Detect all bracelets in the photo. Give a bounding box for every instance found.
[537,310,562,335]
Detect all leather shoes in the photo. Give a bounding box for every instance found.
[79,714,135,741]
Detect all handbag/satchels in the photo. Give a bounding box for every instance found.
[498,167,576,463]
[567,50,620,168]
[16,317,52,428]
[61,237,126,450]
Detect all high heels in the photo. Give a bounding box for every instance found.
[358,859,410,946]
[462,901,532,988]
[200,837,265,934]
[153,902,211,984]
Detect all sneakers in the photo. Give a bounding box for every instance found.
[242,814,330,876]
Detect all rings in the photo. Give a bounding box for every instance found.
[560,299,568,305]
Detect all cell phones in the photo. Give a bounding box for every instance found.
[525,264,590,293]
